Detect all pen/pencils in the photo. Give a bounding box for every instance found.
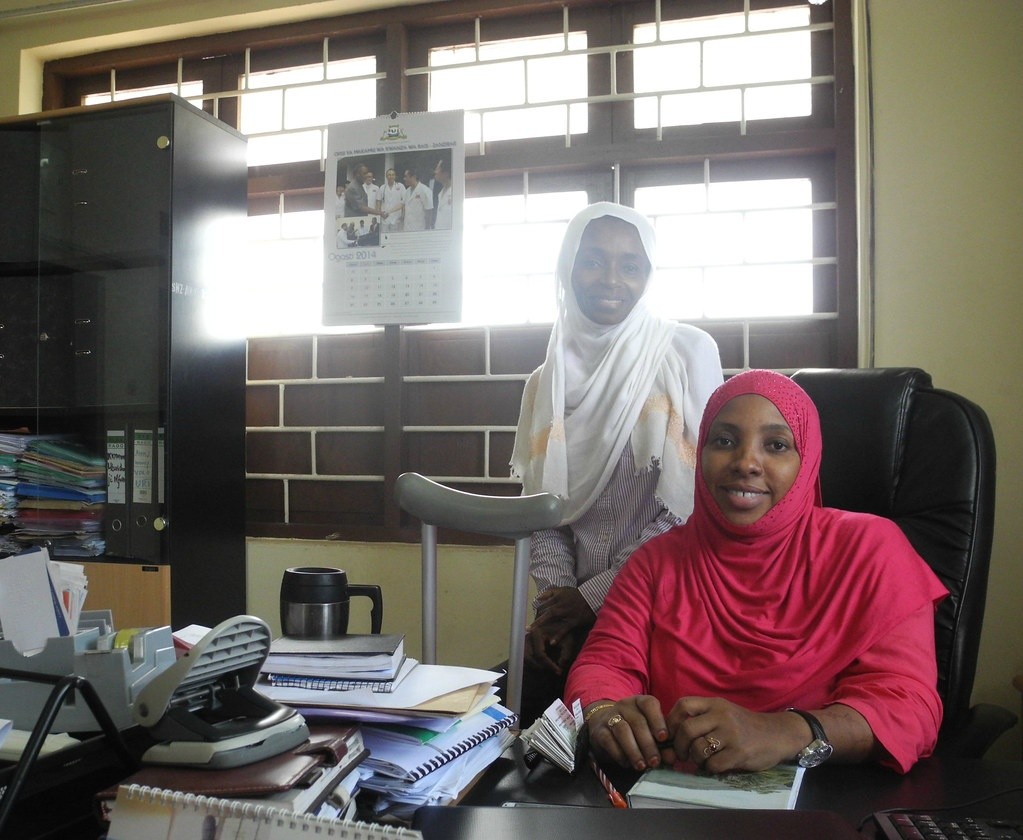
[591,758,626,808]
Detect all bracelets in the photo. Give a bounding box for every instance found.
[584,705,614,723]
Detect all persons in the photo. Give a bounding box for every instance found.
[335,217,380,247]
[509,202,726,733]
[563,368,951,775]
[336,159,451,229]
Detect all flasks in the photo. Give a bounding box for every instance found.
[280,566,383,636]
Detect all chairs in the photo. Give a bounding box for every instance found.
[791,366,998,738]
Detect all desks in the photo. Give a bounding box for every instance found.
[455,735,1023,840]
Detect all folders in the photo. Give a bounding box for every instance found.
[106,422,168,566]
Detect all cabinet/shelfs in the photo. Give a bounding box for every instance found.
[0,92,246,639]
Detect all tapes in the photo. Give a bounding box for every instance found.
[114,629,139,654]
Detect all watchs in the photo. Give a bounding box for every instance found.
[785,708,833,769]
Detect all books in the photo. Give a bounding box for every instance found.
[95,623,521,840]
[626,764,805,810]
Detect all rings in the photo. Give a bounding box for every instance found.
[704,735,721,755]
[608,715,624,730]
